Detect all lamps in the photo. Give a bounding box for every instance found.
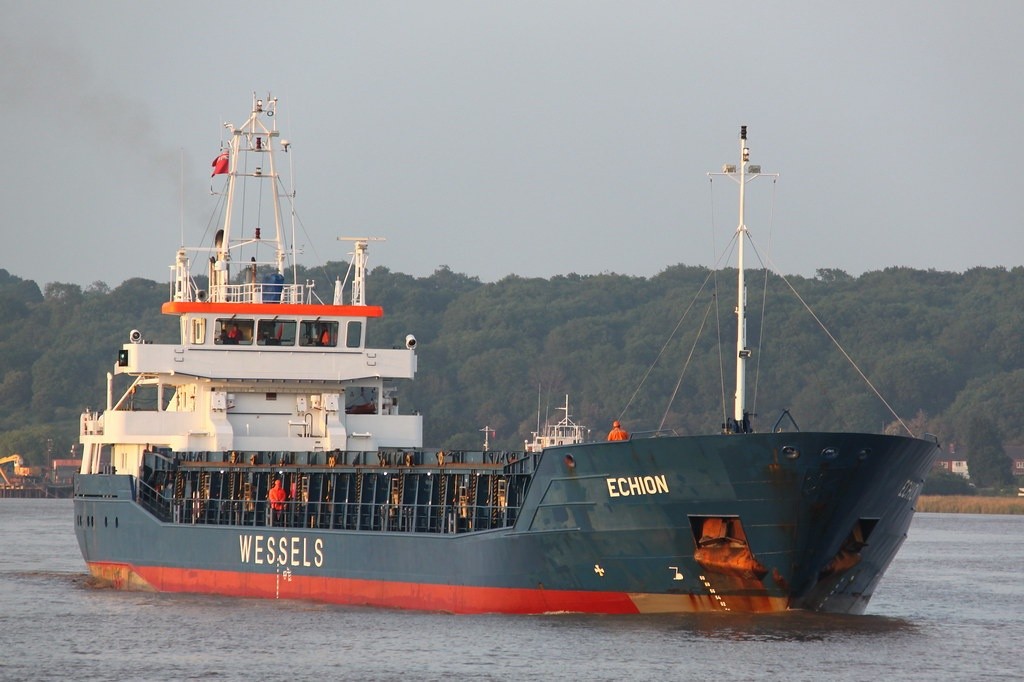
[405,334,418,352]
[128,329,142,343]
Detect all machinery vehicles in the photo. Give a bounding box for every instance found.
[0,453,41,485]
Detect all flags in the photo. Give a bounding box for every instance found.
[211,152,230,177]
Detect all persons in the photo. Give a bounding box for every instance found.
[228,324,244,340]
[607,421,629,441]
[268,478,286,520]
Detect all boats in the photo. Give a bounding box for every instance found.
[523,393,591,452]
[73,86,939,614]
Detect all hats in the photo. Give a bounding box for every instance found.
[613,421,619,427]
[275,479,281,485]
